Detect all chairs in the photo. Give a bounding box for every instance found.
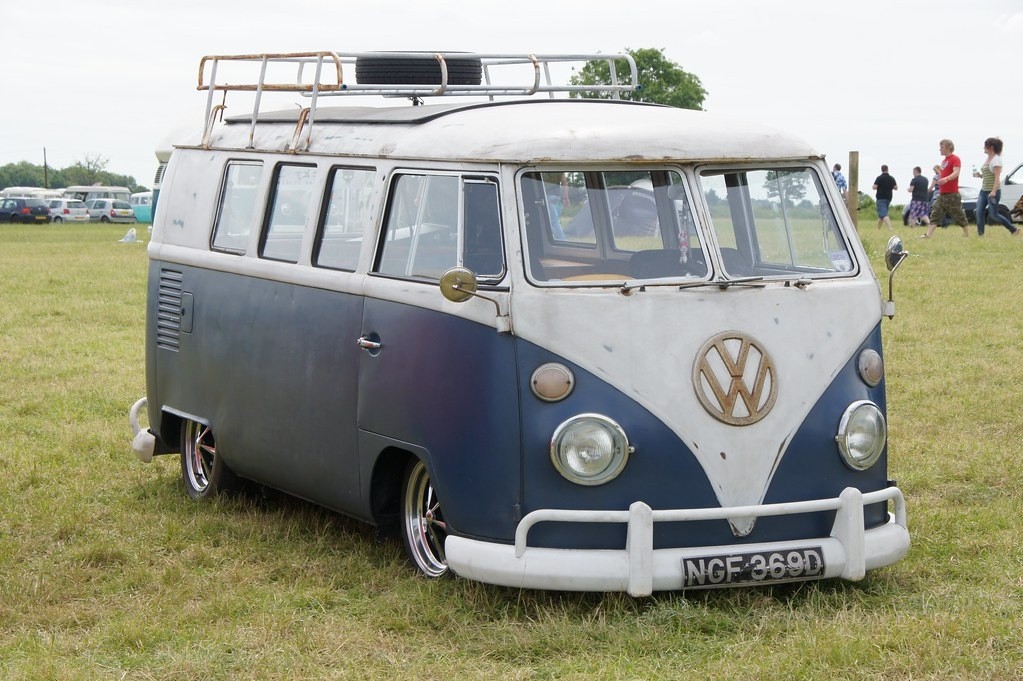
[630,248,746,277]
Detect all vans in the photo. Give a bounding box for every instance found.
[61,184,132,203]
[127,50,910,600]
[131,191,153,204]
[0,187,66,199]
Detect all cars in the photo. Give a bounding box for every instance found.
[84,198,136,224]
[900,185,981,226]
[273,194,299,219]
[45,198,91,225]
[0,197,53,225]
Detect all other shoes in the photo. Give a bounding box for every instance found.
[920,234,928,239]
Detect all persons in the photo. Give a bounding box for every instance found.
[928,165,948,227]
[872,165,898,230]
[907,166,930,226]
[831,164,848,201]
[543,171,570,239]
[921,139,969,238]
[973,138,1021,236]
